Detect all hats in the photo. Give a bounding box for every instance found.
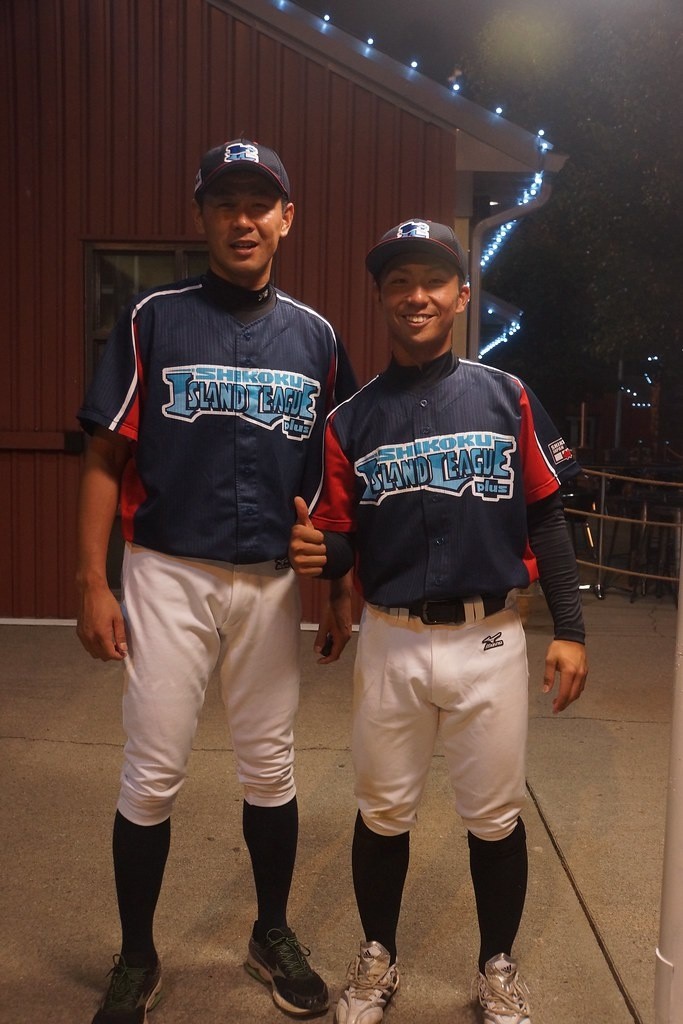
[193,136,291,205]
[364,217,470,285]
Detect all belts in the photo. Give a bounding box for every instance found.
[406,593,505,627]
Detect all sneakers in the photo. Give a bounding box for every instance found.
[478,952,531,1024]
[247,920,330,1016]
[92,944,163,1024]
[335,941,402,1024]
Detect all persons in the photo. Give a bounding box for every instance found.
[74,138,360,1024]
[287,218,589,1024]
